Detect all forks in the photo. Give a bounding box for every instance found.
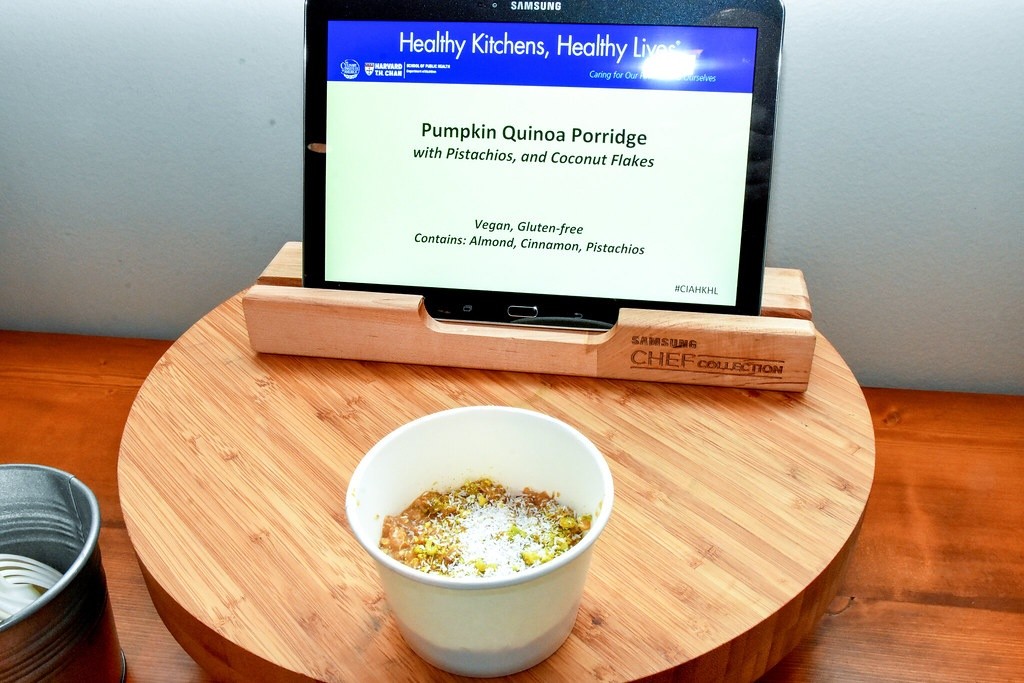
[0,553,63,624]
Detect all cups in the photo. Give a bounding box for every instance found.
[0,462,127,683]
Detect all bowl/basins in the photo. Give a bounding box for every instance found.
[345,405,615,678]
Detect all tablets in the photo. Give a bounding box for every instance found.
[301,0,786,333]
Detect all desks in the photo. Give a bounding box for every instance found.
[117,287,877,683]
